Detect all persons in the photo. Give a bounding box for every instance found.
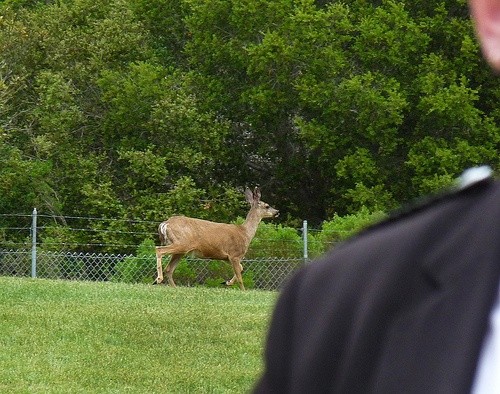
[250,2,500,394]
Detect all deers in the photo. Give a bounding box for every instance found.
[151,186,280,292]
